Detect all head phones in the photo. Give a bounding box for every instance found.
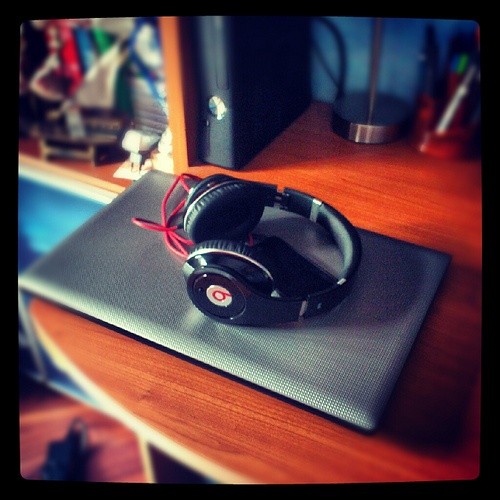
[178,172,364,328]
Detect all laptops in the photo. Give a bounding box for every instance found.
[17,168,452,436]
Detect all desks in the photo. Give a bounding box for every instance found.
[28,95,485,486]
